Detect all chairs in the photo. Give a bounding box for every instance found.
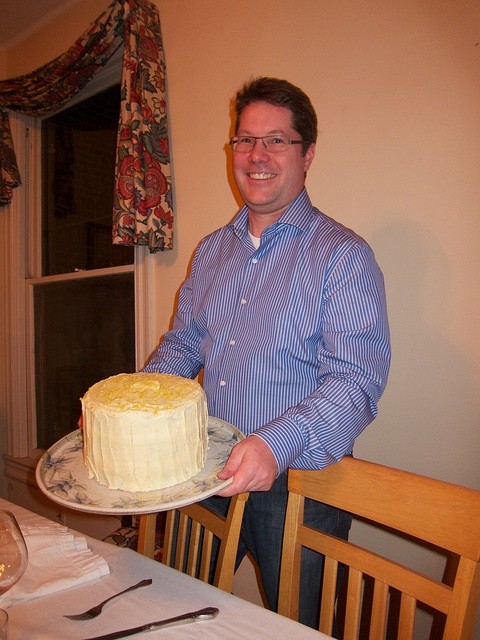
[277,455,480,640]
[137,492,250,594]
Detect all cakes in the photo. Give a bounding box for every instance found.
[79,372,209,494]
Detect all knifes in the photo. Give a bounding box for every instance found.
[89,608,217,639]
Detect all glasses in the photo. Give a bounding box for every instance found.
[231,136,308,152]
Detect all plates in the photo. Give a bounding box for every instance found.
[35,415,247,516]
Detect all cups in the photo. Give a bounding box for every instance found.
[0,609,9,639]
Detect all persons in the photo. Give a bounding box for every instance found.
[76,77,392,628]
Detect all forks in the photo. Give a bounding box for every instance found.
[62,579,152,620]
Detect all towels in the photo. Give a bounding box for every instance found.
[0,524,111,609]
